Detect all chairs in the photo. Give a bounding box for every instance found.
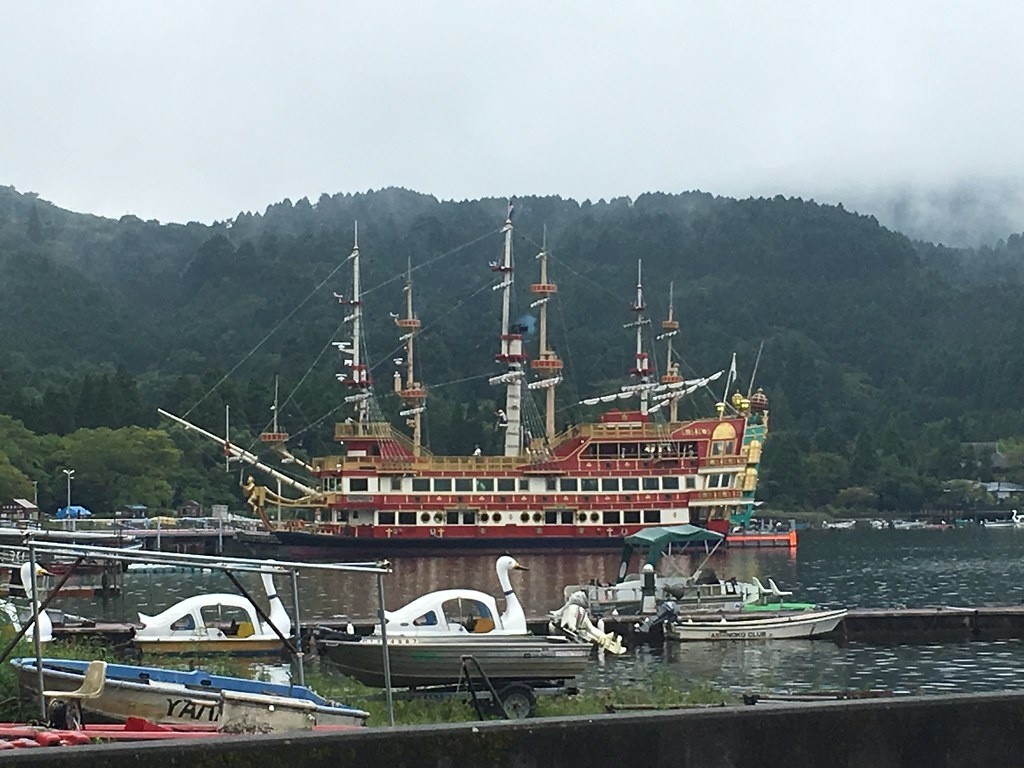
[43,659,107,731]
[475,619,494,633]
[228,621,254,638]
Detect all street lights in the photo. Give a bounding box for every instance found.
[63,469,75,531]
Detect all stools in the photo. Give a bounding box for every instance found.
[189,700,218,721]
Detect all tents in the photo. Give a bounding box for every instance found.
[55,506,91,520]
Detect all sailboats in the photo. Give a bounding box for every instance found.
[151,192,799,552]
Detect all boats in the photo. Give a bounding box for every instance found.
[819,509,1024,530]
[1,560,56,644]
[46,531,144,572]
[632,598,849,644]
[11,653,371,738]
[313,623,594,689]
[532,523,793,615]
[131,558,293,655]
[374,554,530,642]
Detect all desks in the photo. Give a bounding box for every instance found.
[220,689,316,740]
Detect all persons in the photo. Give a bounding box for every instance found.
[688,447,695,457]
[472,444,482,456]
[653,446,678,458]
[240,476,258,514]
[494,408,507,432]
[523,427,535,449]
